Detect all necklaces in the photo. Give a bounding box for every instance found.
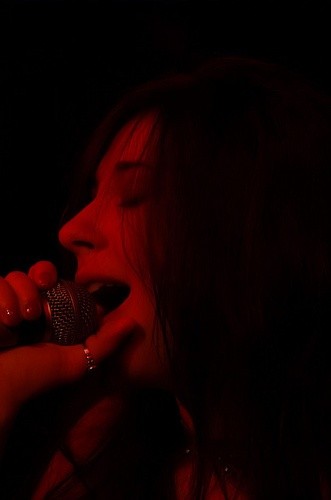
[176,414,245,474]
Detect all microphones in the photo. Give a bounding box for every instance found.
[0,278,97,354]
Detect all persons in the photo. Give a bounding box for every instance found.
[0,57,331,499]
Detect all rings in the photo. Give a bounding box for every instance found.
[82,340,98,374]
